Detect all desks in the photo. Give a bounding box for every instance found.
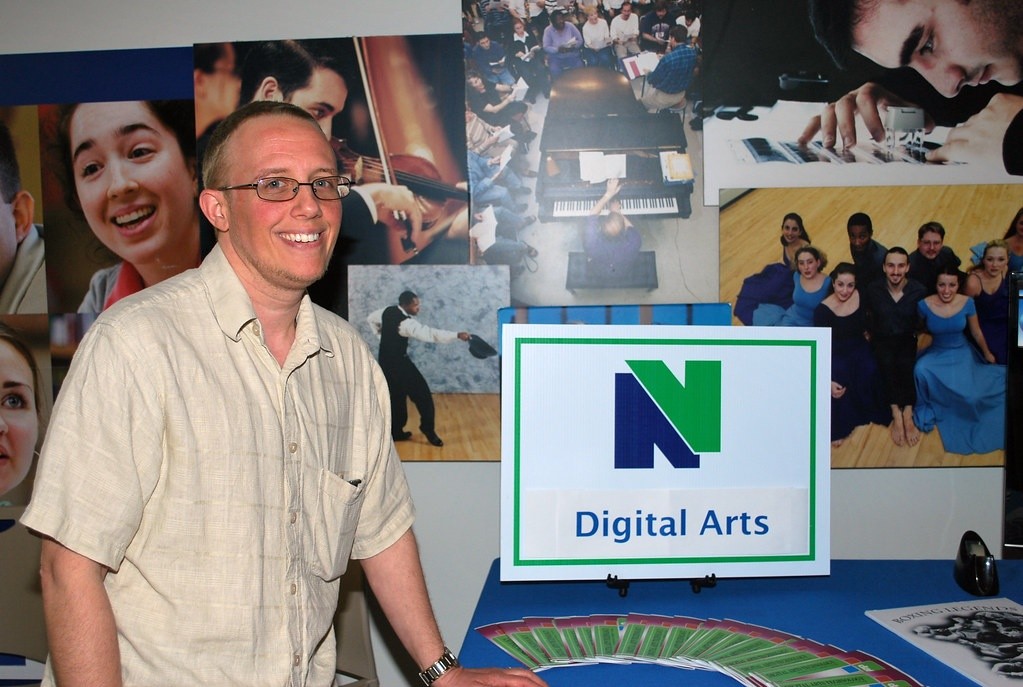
[457,555,1023,687]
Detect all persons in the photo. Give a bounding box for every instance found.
[196,40,467,310]
[734,210,1023,456]
[0,320,41,507]
[367,291,470,445]
[19,102,547,686]
[462,0,701,271]
[0,121,48,315]
[799,0,1023,174]
[59,97,200,314]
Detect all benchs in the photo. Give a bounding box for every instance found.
[565,250,658,293]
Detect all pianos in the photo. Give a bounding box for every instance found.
[533,111,695,224]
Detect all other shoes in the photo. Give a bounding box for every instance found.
[520,216,535,231]
[543,87,551,99]
[512,203,526,214]
[528,92,536,103]
[512,186,531,194]
[394,431,411,441]
[522,241,537,257]
[522,169,537,178]
[419,426,443,445]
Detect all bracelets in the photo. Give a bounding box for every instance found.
[418,647,457,686]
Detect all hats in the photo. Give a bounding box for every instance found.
[466,335,496,360]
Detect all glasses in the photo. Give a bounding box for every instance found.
[217,177,352,201]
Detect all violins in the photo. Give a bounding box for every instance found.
[325,135,470,233]
